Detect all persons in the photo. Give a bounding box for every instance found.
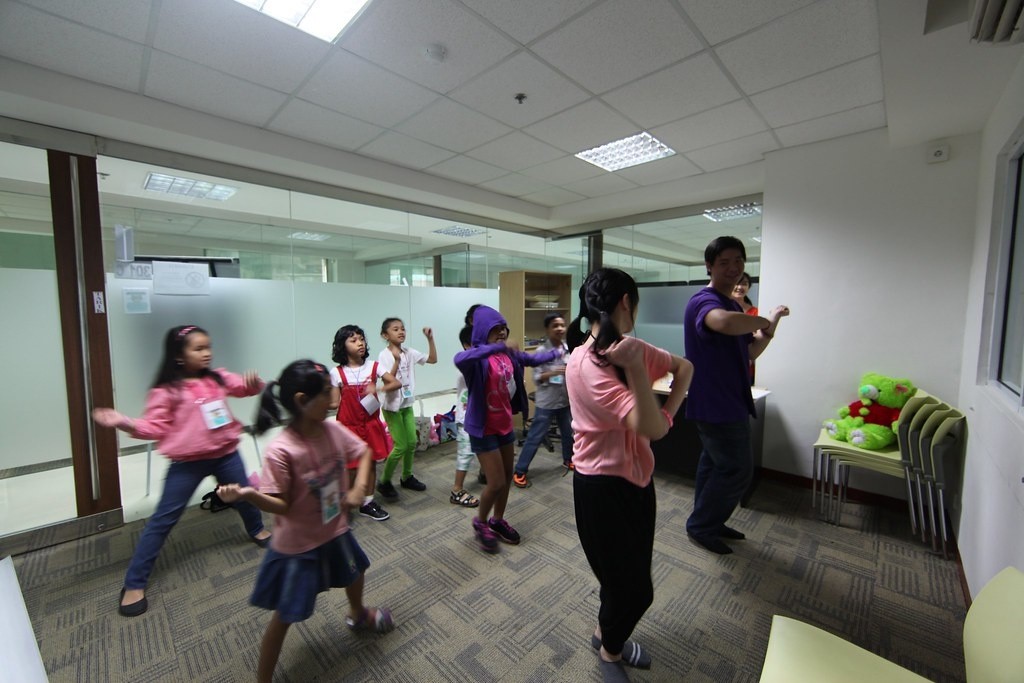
[454,306,568,552]
[376,317,439,501]
[327,325,402,530]
[449,304,490,508]
[683,236,789,556]
[564,269,693,683]
[91,325,273,617]
[513,312,576,489]
[731,272,758,387]
[215,359,394,683]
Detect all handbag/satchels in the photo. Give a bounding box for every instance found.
[414,396,431,451]
[434,406,457,443]
[429,423,440,447]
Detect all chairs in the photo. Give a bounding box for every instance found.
[813,388,967,561]
[758,567,1024,683]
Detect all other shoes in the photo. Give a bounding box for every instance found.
[120,587,148,616]
[252,533,272,547]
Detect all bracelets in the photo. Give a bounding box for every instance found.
[660,408,673,429]
[762,320,770,329]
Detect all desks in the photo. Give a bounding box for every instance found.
[652,376,771,507]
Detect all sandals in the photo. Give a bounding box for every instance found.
[478,469,488,484]
[450,491,480,507]
[347,606,392,633]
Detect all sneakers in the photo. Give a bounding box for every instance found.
[513,471,527,488]
[563,460,575,470]
[400,474,426,491]
[359,498,390,521]
[375,480,399,497]
[473,516,498,554]
[488,517,520,544]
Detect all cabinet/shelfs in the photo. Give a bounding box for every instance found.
[499,271,573,431]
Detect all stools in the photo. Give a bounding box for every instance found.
[518,391,576,452]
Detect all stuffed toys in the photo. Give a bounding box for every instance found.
[823,373,917,450]
